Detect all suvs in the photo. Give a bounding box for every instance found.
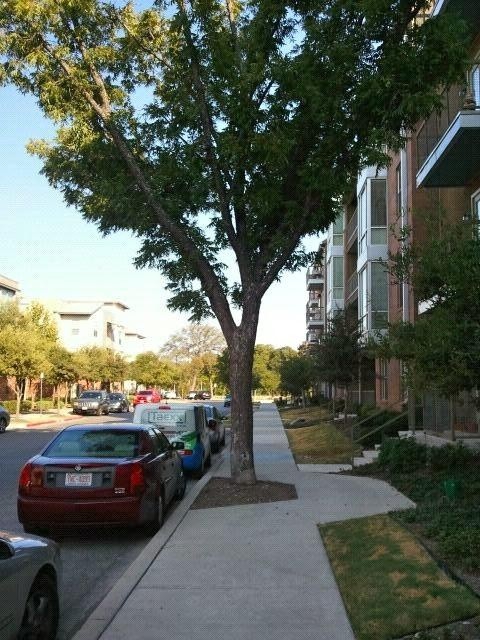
[72,390,111,416]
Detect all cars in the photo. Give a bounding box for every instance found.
[0,528,64,640]
[163,390,177,399]
[0,404,11,434]
[194,391,212,400]
[223,392,232,407]
[201,404,228,454]
[132,389,162,407]
[16,421,188,538]
[108,392,130,413]
[186,391,198,400]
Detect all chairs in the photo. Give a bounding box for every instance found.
[113,443,133,450]
[59,442,79,453]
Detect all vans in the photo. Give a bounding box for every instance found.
[131,402,218,480]
